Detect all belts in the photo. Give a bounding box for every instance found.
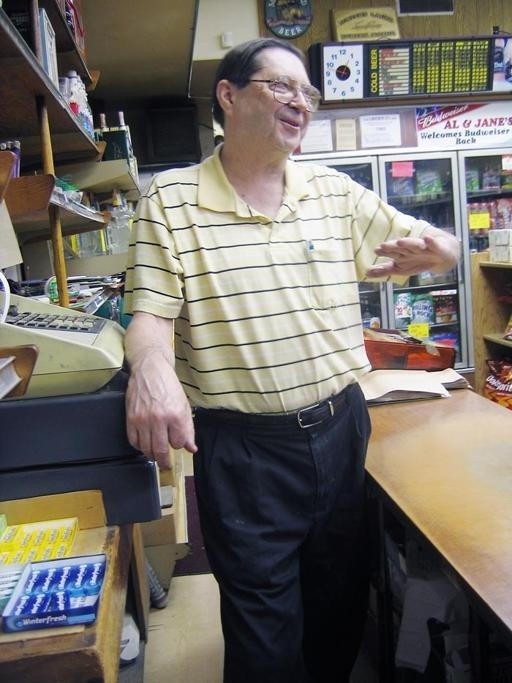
[190,385,350,434]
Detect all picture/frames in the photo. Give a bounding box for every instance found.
[264,1,312,40]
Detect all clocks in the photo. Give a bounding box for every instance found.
[320,42,365,104]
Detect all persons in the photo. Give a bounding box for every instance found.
[122,38,460,683]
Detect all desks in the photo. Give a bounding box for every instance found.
[1,521,149,683]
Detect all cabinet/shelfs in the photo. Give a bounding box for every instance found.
[451,146,512,414]
[0,1,191,589]
[296,148,474,376]
[358,380,511,681]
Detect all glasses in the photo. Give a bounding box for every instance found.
[247,76,322,114]
